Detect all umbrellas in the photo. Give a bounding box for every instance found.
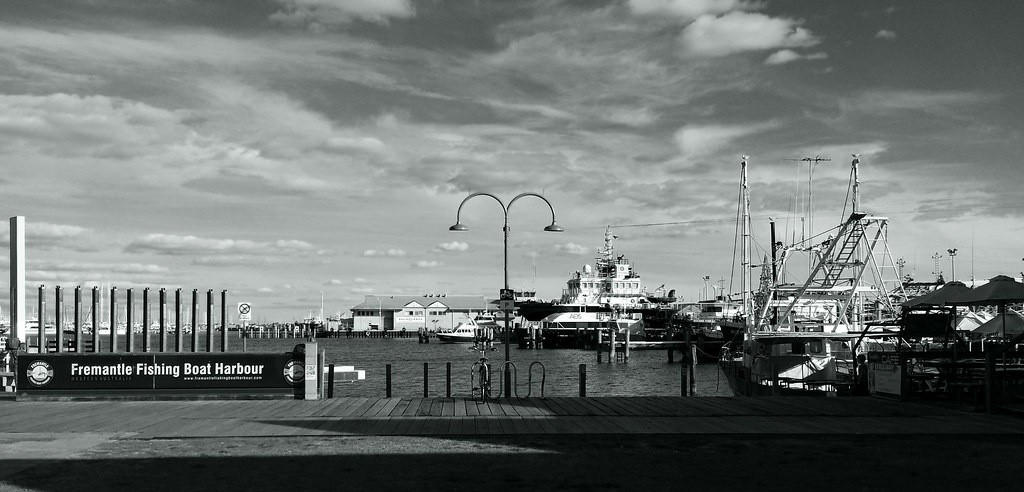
[901,280,976,366]
[944,275,1024,373]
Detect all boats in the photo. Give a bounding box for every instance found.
[436,302,505,343]
[516,145,945,397]
[0,300,266,336]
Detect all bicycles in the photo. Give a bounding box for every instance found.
[468,345,497,404]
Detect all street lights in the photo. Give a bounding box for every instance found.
[319,292,324,326]
[448,192,566,398]
[947,248,958,282]
[703,276,710,301]
[377,298,385,330]
